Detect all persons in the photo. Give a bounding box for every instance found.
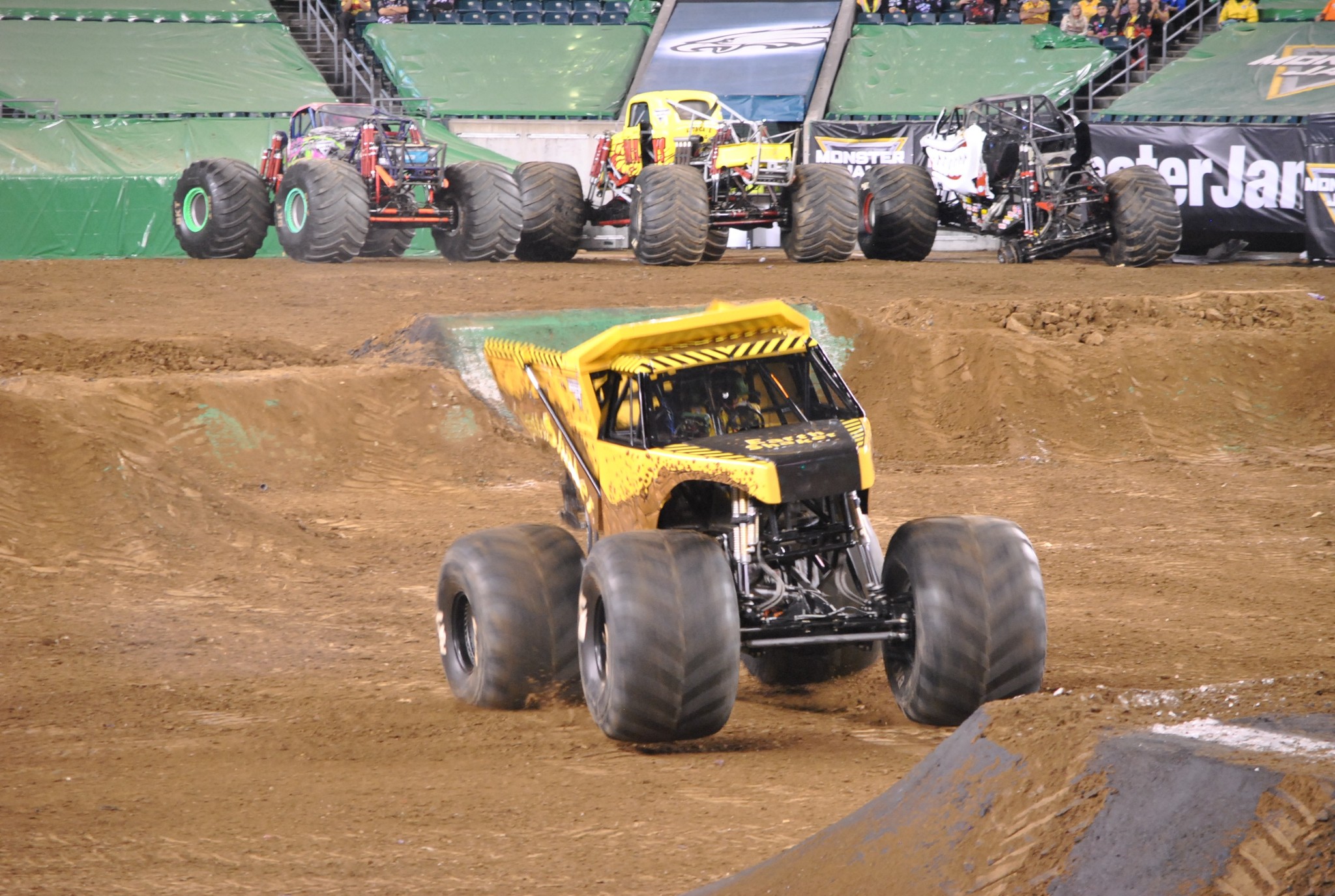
[336,0,454,42]
[687,370,779,535]
[1315,0,1335,21]
[1219,0,1259,23]
[858,0,1216,69]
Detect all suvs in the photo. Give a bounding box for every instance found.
[172,101,524,265]
[856,91,1185,268]
[510,89,860,267]
[434,294,1048,746]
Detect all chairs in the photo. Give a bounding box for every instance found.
[352,0,1074,24]
